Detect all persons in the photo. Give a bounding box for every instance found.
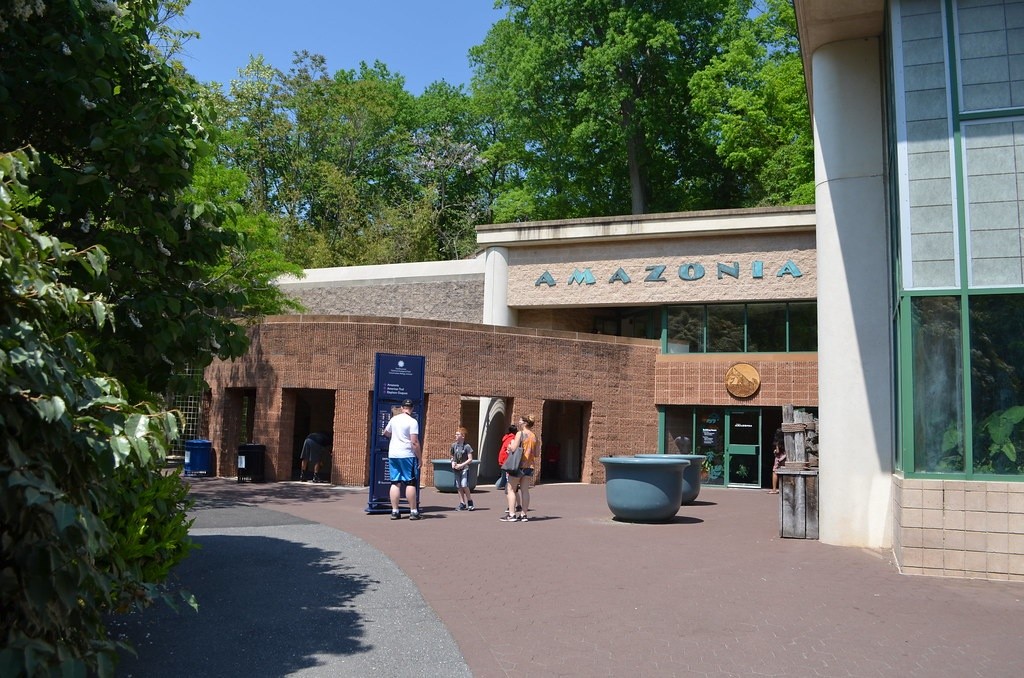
[766,428,785,494]
[498,425,522,512]
[498,414,539,522]
[449,427,475,511]
[382,399,425,519]
[298,431,333,483]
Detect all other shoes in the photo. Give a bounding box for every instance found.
[768,490,779,494]
[504,504,522,514]
[299,476,307,482]
[313,478,323,483]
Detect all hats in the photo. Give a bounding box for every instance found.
[402,399,413,406]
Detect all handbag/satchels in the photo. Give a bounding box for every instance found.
[501,432,523,472]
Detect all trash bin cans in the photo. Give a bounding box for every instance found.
[238,443,265,483]
[184,440,212,477]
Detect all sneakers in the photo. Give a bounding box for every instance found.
[517,513,528,522]
[454,503,467,511]
[467,500,474,510]
[391,511,402,520]
[409,512,423,520]
[499,514,517,522]
